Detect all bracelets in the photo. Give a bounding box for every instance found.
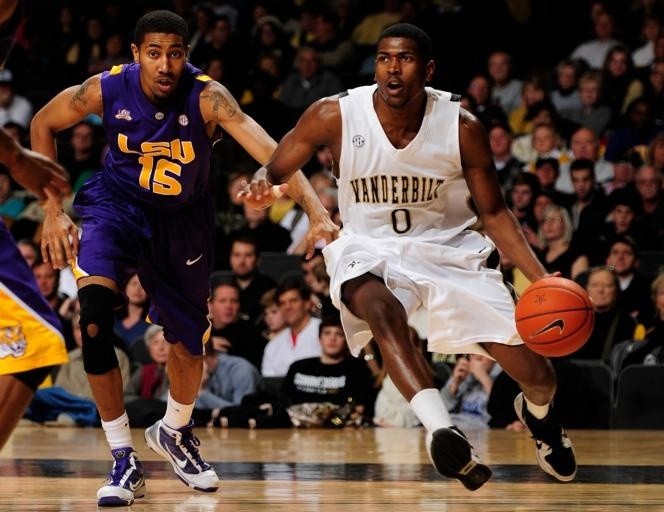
[364,354,374,361]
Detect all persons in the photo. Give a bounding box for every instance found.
[30,9,337,507]
[239,23,577,491]
[2,2,664,431]
[2,1,72,453]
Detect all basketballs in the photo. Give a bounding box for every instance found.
[516,277,594,357]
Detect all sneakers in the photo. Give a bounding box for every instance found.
[97,447,145,506]
[513,391,577,482]
[432,426,491,491]
[144,418,220,492]
